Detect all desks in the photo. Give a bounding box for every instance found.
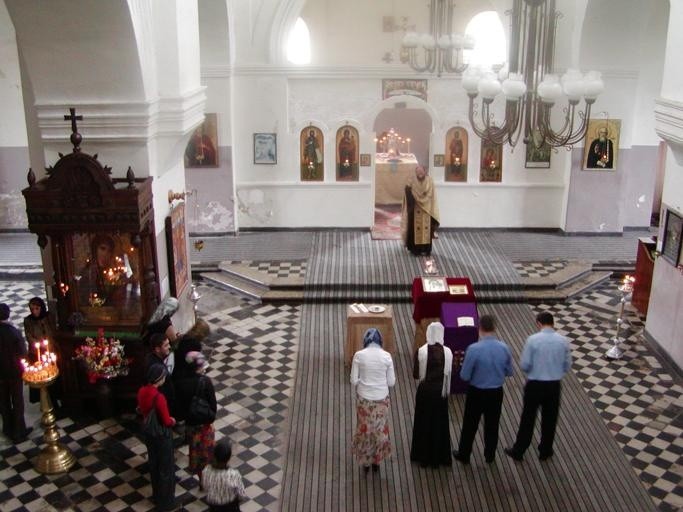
[376,153,418,204]
[344,303,394,368]
[410,277,479,324]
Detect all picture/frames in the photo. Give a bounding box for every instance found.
[360,154,370,166]
[658,208,683,268]
[381,78,428,103]
[581,118,623,170]
[166,202,189,299]
[186,112,220,168]
[253,132,278,165]
[434,154,444,166]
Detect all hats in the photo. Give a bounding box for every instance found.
[185,351,209,370]
[147,364,166,382]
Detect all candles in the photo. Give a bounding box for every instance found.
[19,339,55,381]
[375,128,411,153]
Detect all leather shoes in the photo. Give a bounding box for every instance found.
[452,449,469,464]
[505,447,523,460]
[540,449,553,460]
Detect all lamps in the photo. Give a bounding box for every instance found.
[402,1,469,75]
[462,0,604,149]
[605,274,635,358]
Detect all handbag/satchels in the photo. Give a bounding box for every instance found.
[143,393,171,438]
[188,396,211,421]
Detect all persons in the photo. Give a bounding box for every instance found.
[411,321,456,469]
[0,302,33,444]
[400,164,440,258]
[452,314,513,463]
[136,296,251,512]
[186,124,215,167]
[504,311,572,462]
[587,125,614,170]
[482,147,495,171]
[339,129,356,179]
[350,328,396,473]
[450,131,464,176]
[77,234,136,307]
[303,129,322,180]
[25,297,59,405]
